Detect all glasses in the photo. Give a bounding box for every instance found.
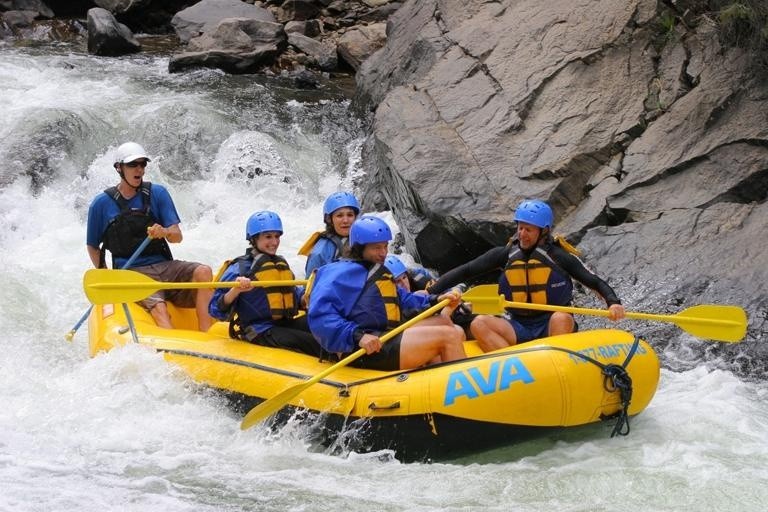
[121,161,148,168]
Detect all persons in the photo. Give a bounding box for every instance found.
[304,192,360,279]
[424,200,625,352]
[86,142,215,332]
[210,212,324,359]
[309,216,466,370]
[381,254,473,321]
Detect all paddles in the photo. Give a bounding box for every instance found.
[241,292,459,430]
[83,269,316,304]
[497,294,747,342]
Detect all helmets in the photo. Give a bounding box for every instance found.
[112,141,151,167]
[384,253,408,279]
[323,190,360,222]
[348,215,392,252]
[245,210,283,241]
[513,199,553,232]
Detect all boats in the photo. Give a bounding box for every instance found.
[86,294,662,465]
